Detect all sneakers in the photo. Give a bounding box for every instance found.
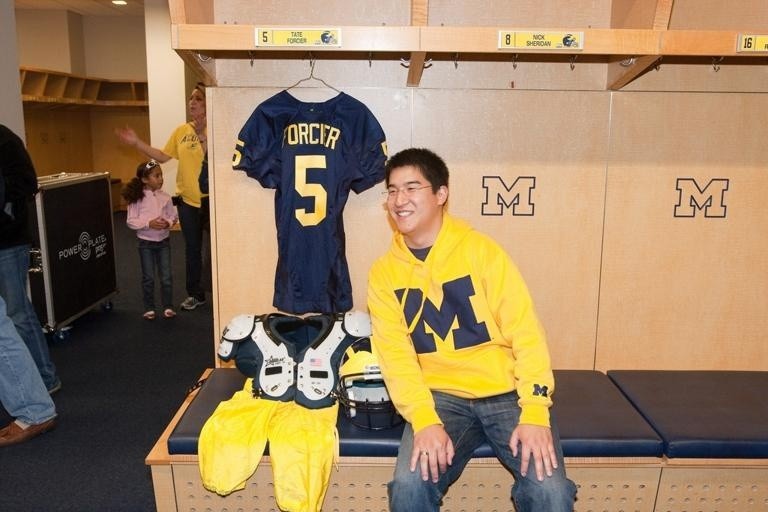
[144,308,176,319]
[180,296,207,310]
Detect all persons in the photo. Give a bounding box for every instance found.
[112,77,220,311]
[0,123,63,396]
[0,295,59,447]
[120,158,181,320]
[367,147,578,511]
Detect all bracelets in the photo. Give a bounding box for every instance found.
[199,136,207,144]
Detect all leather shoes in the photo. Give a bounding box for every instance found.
[0,419,57,446]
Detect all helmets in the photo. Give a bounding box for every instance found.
[337,337,404,430]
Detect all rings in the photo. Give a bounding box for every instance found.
[420,450,429,457]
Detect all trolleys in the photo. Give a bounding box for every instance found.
[21,170,121,342]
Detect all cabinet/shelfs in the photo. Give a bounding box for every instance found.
[20,67,151,110]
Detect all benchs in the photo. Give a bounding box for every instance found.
[141,364,662,512]
[607,358,768,512]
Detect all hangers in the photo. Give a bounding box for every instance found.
[283,51,343,102]
